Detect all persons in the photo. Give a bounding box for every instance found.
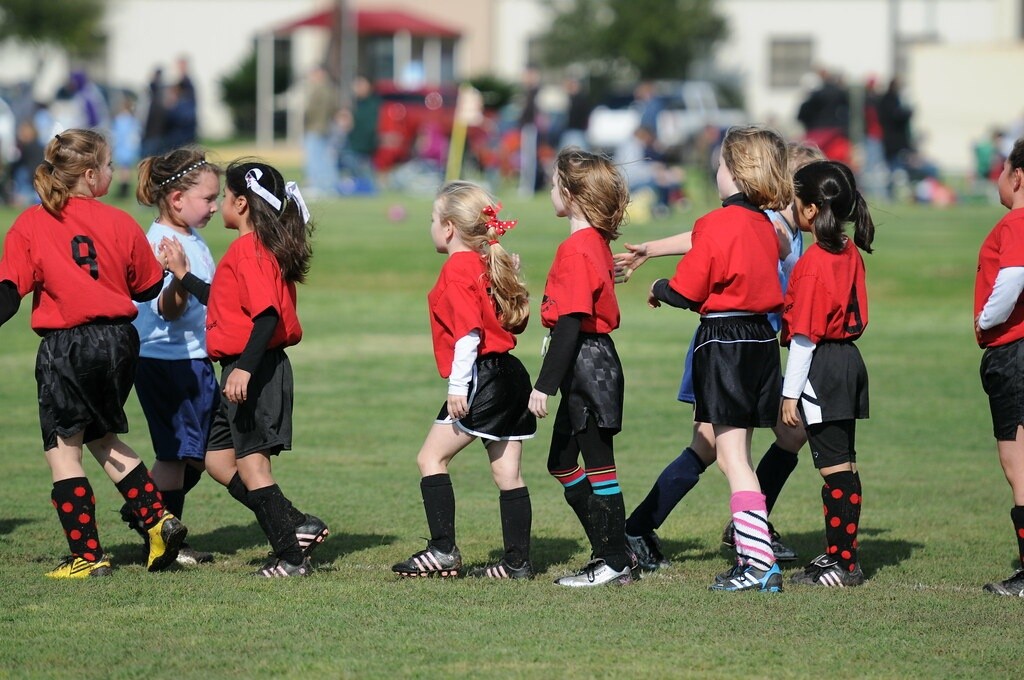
[972,139,1024,597]
[528,150,640,587]
[0,129,187,578]
[392,181,536,583]
[159,162,330,576]
[0,48,198,209]
[646,126,792,593]
[779,159,875,588]
[118,148,225,565]
[519,65,722,226]
[615,140,828,567]
[798,69,914,197]
[302,67,380,194]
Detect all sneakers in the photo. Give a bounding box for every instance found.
[391,536,463,578]
[553,558,632,591]
[293,513,330,559]
[708,561,783,595]
[721,521,799,564]
[789,553,865,589]
[624,520,673,572]
[42,553,114,583]
[246,553,312,585]
[983,572,1024,598]
[570,545,640,576]
[118,503,145,540]
[466,558,529,582]
[146,510,188,572]
[174,543,215,567]
[714,561,759,581]
